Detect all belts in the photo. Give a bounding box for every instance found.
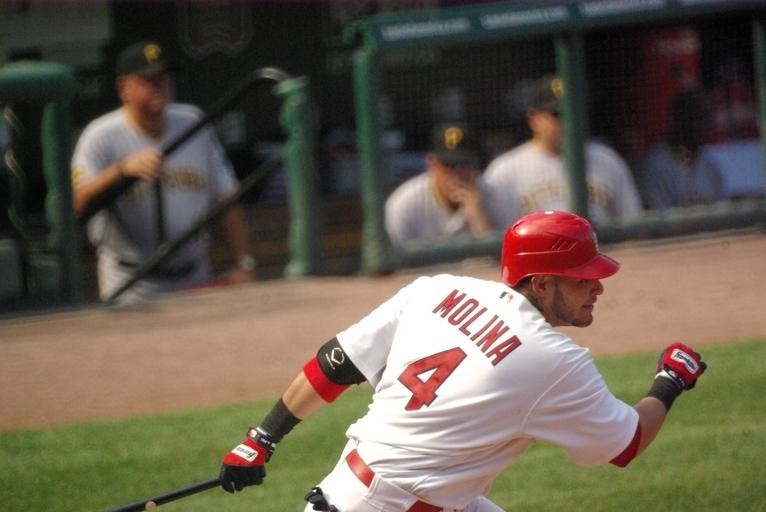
[346,448,442,512]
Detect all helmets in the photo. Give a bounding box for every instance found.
[501,210,621,287]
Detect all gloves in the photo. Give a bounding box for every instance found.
[654,341,707,392]
[220,426,278,494]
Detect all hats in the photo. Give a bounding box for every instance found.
[530,74,564,113]
[116,41,172,80]
[431,120,482,167]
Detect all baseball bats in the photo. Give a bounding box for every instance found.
[107,468,265,511]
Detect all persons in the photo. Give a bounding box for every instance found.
[639,96,728,211]
[68,41,258,308]
[217,211,707,512]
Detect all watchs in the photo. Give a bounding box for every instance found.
[237,255,258,276]
